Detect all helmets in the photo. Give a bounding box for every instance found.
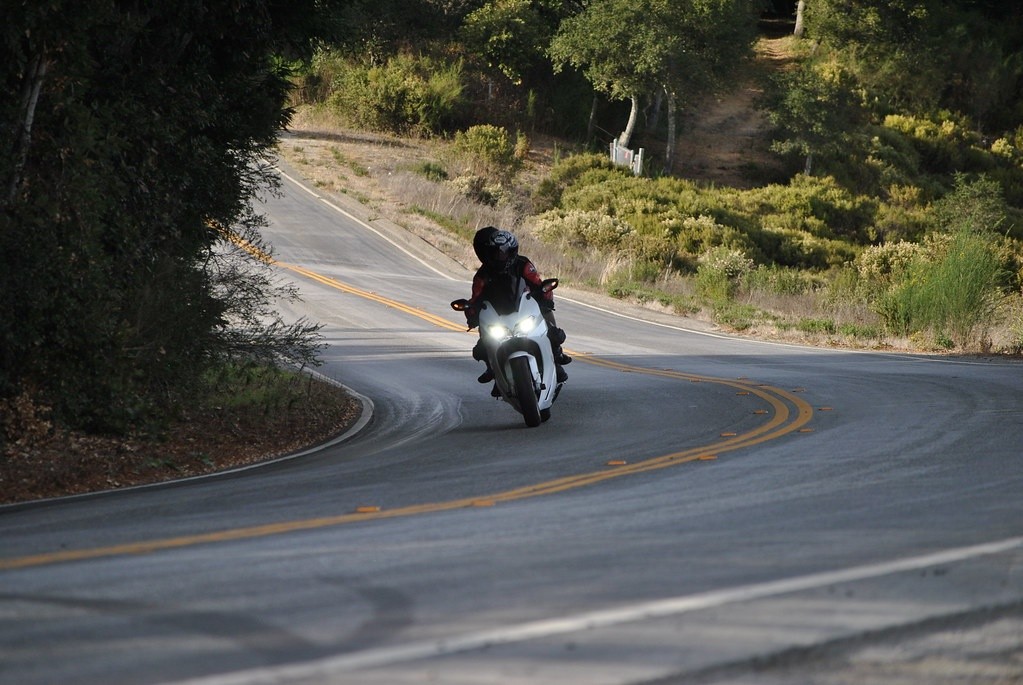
[474,226,500,262]
[485,230,519,268]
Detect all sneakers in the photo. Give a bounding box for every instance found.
[557,352,571,364]
[490,383,502,397]
[556,365,568,382]
[477,366,493,383]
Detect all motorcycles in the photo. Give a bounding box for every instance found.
[451,278,571,428]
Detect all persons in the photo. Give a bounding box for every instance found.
[464,227,573,397]
